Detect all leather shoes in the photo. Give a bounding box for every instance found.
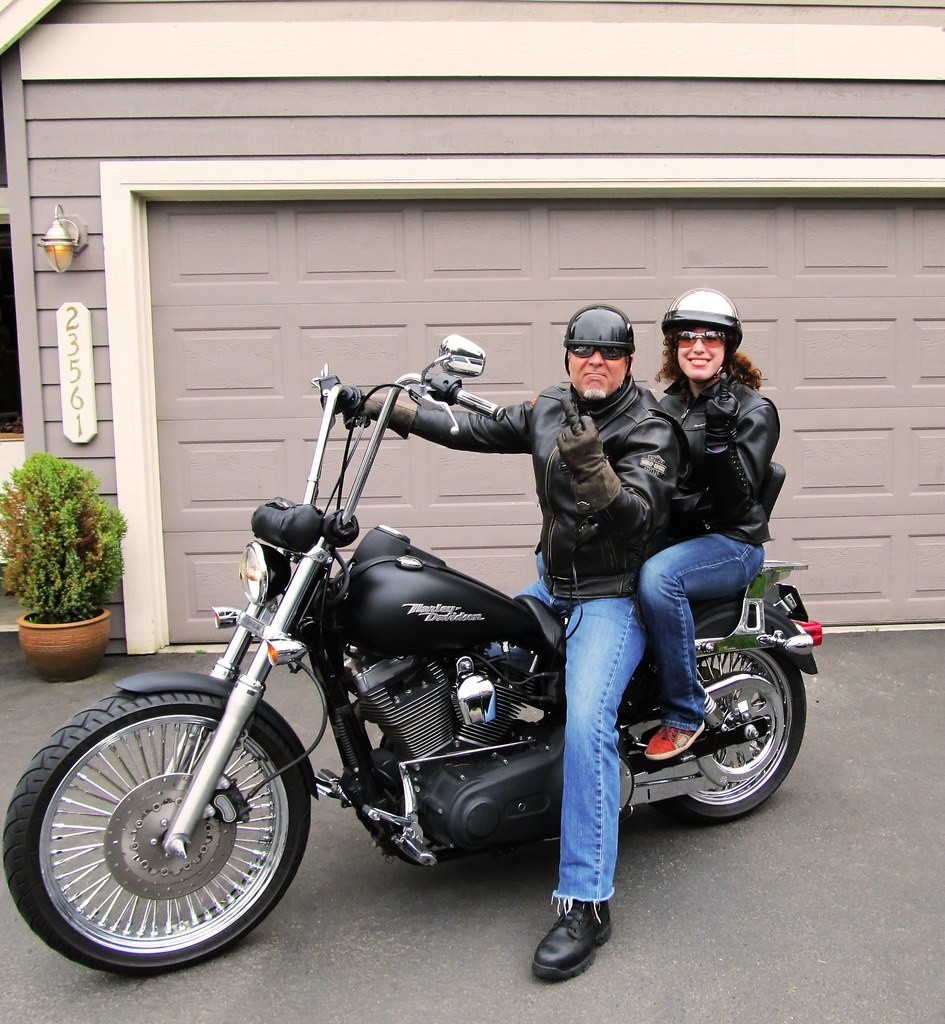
[532,898,612,981]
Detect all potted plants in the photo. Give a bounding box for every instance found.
[0,453,128,682]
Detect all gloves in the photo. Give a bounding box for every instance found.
[557,399,622,515]
[705,373,740,450]
[345,389,418,439]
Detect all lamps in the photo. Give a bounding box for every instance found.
[36,204,88,273]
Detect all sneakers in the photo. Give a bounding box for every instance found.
[644,719,705,760]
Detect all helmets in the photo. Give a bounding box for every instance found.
[563,303,635,351]
[662,288,743,340]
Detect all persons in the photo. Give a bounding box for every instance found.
[342,304,680,982]
[532,288,779,760]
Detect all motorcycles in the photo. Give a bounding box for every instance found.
[3,334,824,979]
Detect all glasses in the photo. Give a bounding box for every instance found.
[567,344,629,360]
[675,330,726,349]
